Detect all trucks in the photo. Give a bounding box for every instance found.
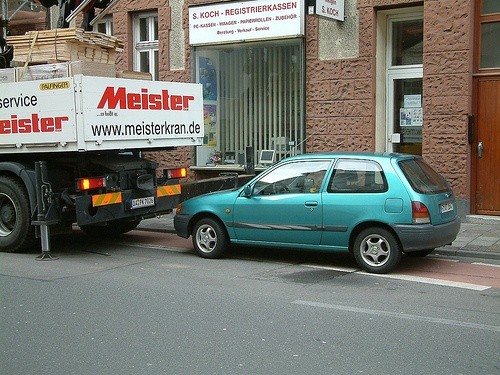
[0,74,204,261]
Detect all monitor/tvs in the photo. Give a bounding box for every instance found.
[259,150,275,163]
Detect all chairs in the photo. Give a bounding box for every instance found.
[333,168,360,190]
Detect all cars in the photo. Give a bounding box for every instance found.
[174,153,461,274]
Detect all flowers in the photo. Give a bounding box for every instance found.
[209,150,222,163]
